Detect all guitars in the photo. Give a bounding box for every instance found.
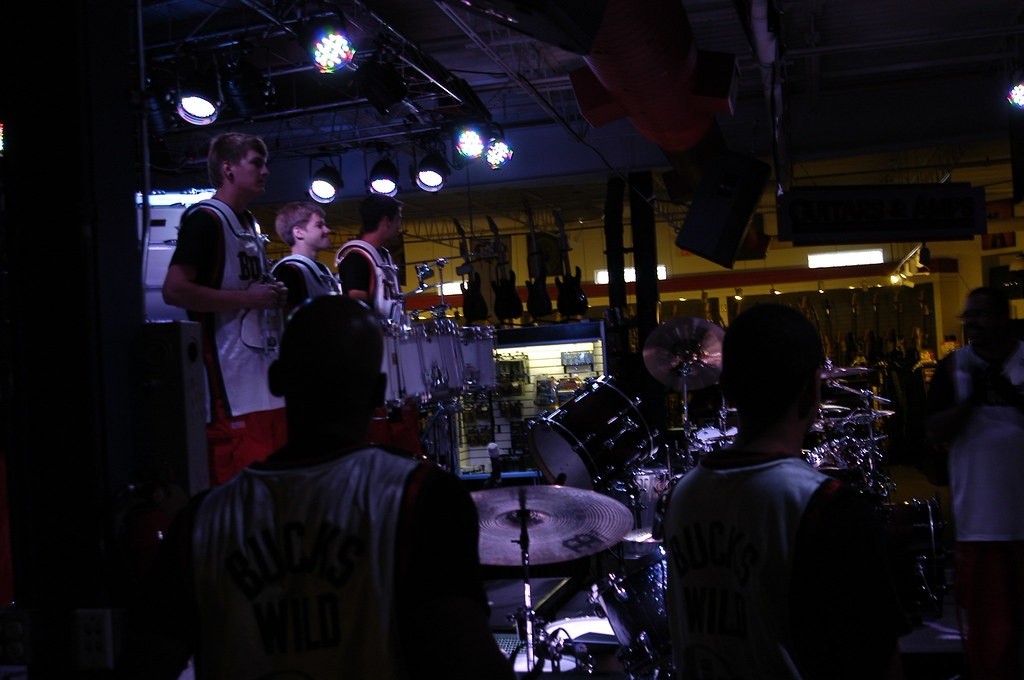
[626,287,939,384]
[453,199,588,322]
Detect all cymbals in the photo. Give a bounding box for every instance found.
[642,317,727,393]
[469,485,634,566]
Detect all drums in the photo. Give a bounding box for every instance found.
[503,651,584,680]
[527,375,659,490]
[608,467,669,618]
[883,494,944,613]
[382,317,496,411]
[536,616,629,680]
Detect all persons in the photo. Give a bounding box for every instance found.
[660,302,923,680]
[927,285,1024,680]
[846,326,957,465]
[270,201,342,306]
[334,194,403,407]
[161,133,288,488]
[110,291,519,680]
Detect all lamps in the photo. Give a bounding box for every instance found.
[455,115,514,169]
[362,146,399,198]
[414,142,448,193]
[129,48,221,141]
[307,153,344,205]
[294,4,356,74]
[699,244,932,304]
